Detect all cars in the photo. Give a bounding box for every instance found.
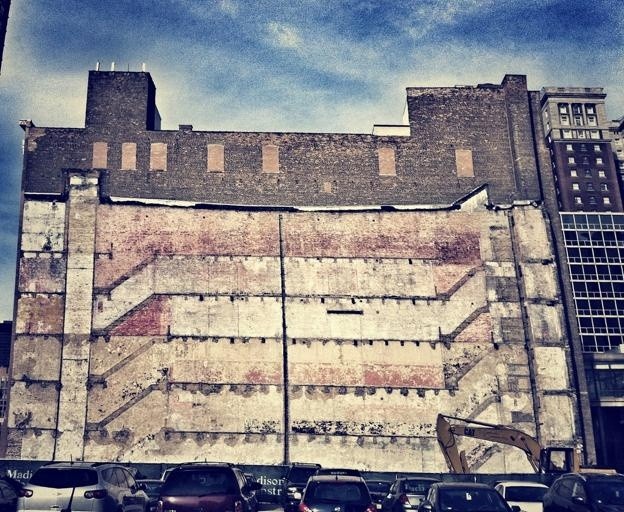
[1,461,262,510]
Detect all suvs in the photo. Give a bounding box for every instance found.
[281,463,323,511]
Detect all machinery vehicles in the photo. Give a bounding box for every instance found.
[436,413,617,480]
